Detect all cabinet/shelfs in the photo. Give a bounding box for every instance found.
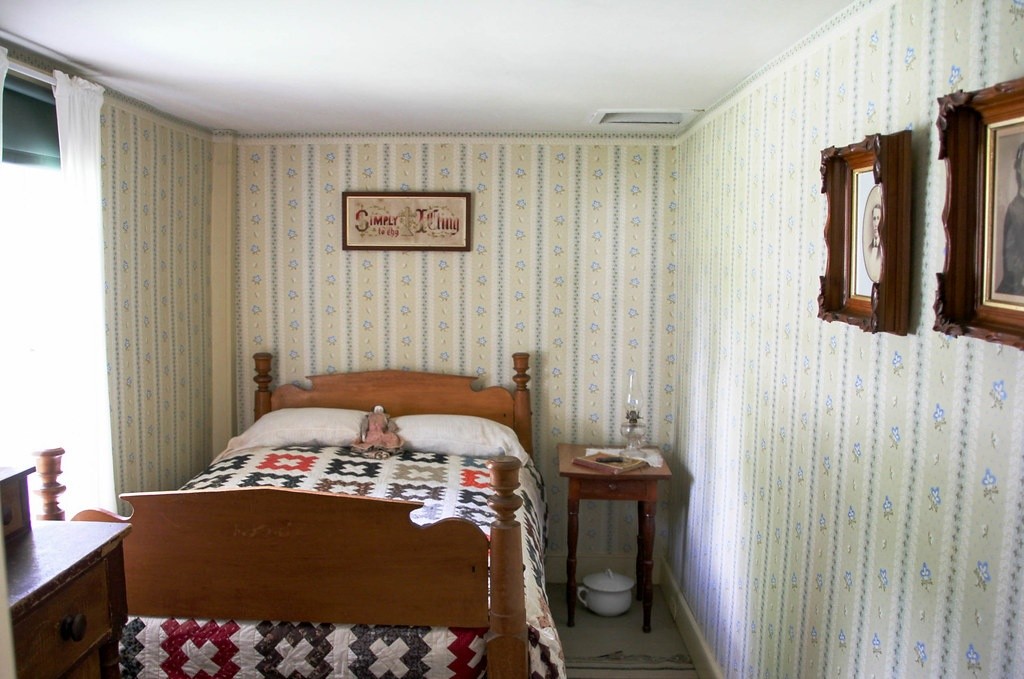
[0,465,133,678]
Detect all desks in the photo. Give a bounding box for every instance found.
[557,442,673,633]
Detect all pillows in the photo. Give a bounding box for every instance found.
[228,407,390,463]
[392,414,528,467]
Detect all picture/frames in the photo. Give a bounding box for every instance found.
[341,191,471,252]
[931,76,1024,351]
[817,130,914,336]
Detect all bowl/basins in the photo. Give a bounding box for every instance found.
[576,568,634,617]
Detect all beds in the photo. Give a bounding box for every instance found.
[35,352,567,679]
